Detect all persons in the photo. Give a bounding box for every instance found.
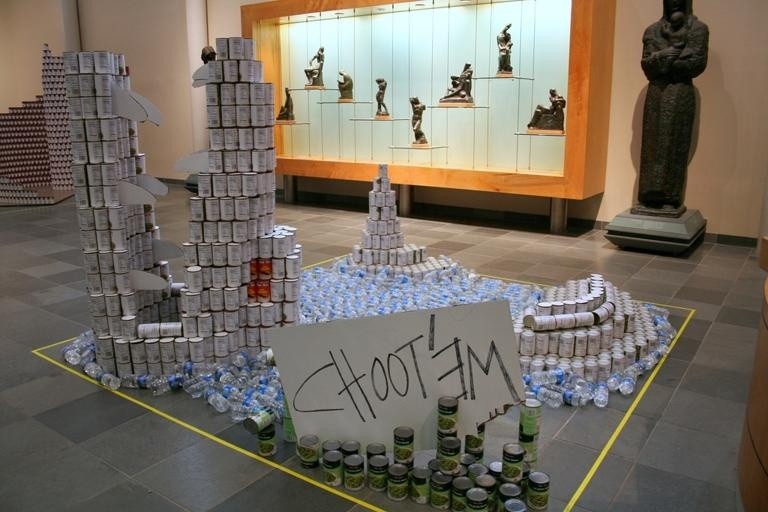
[304,46,325,85]
[336,69,354,100]
[407,95,429,144]
[276,87,296,121]
[438,59,474,104]
[373,77,391,115]
[633,1,709,212]
[527,87,566,132]
[496,22,514,72]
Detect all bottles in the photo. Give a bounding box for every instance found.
[60,255,677,425]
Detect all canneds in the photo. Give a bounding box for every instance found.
[243,389,550,512]
[348,163,450,282]
[513,272,659,378]
[0,36,303,379]
